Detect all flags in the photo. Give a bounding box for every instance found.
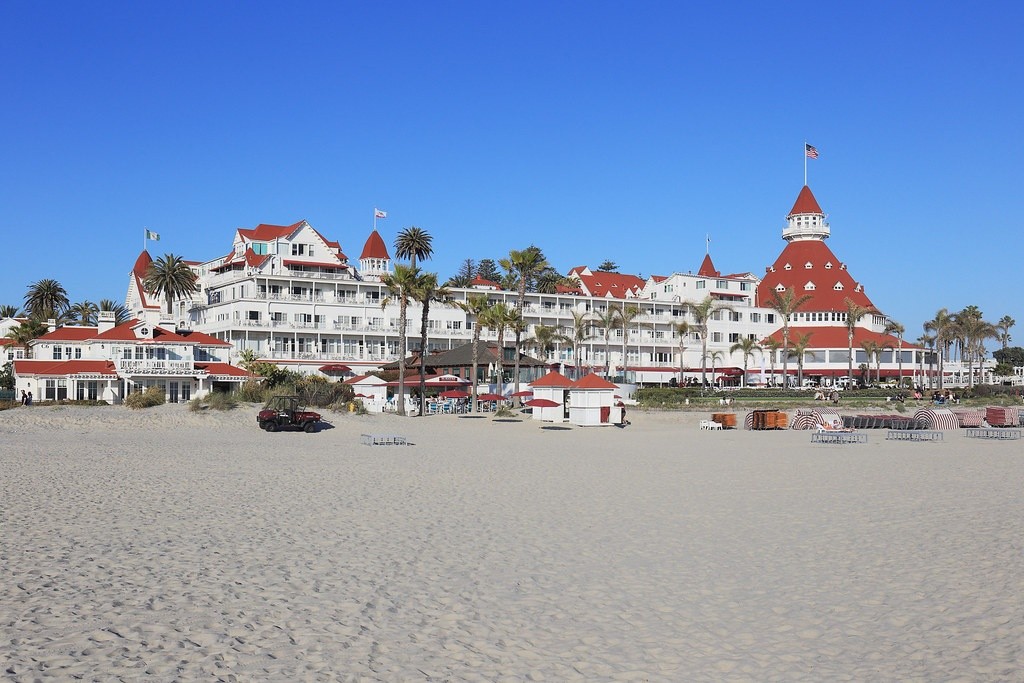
[708,238,711,242]
[805,143,819,160]
[376,210,387,218]
[146,229,160,241]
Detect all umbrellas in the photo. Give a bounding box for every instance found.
[354,394,366,397]
[440,389,472,404]
[525,399,560,423]
[479,393,506,412]
[614,395,622,398]
[510,391,533,401]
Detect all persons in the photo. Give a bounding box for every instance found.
[413,395,489,413]
[617,400,627,423]
[898,391,961,404]
[814,384,839,404]
[27,392,32,405]
[21,390,27,407]
[340,376,344,383]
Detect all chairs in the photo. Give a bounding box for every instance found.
[430,398,496,415]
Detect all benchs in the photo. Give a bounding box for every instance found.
[964,428,1023,441]
[887,430,944,442]
[811,433,867,444]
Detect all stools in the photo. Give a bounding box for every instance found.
[699,420,722,431]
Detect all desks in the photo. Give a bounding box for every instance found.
[710,413,736,430]
[825,430,853,433]
